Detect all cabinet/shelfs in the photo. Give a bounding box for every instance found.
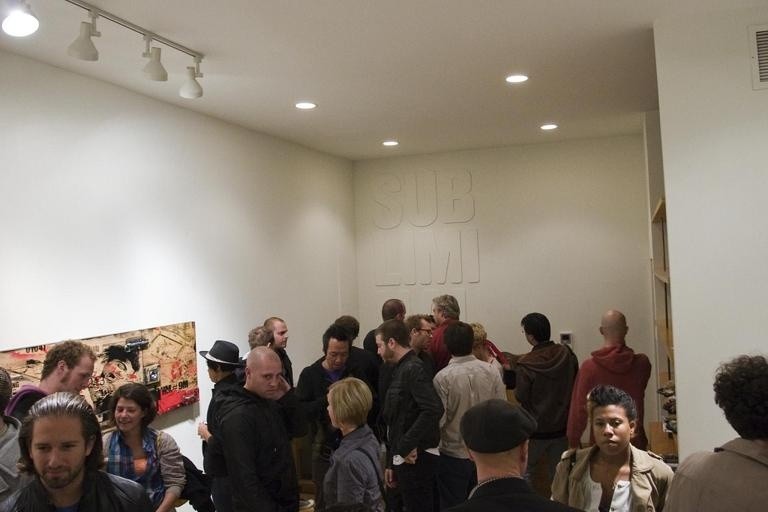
[650,195,677,436]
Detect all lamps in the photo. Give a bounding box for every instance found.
[0,0,205,103]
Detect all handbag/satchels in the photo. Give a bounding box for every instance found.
[153,431,199,512]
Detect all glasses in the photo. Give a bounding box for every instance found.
[416,328,433,337]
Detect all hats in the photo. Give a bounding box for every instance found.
[459,397,539,454]
[199,340,244,366]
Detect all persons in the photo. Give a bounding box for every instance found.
[4,340,97,422]
[432,322,507,512]
[198,317,315,510]
[514,312,579,493]
[550,384,675,512]
[374,320,446,512]
[363,296,503,384]
[0,391,152,512]
[0,367,35,502]
[320,377,384,511]
[443,399,586,512]
[565,310,651,451]
[101,383,186,512]
[661,356,768,512]
[297,315,384,470]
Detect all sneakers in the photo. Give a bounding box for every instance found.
[298,498,315,509]
